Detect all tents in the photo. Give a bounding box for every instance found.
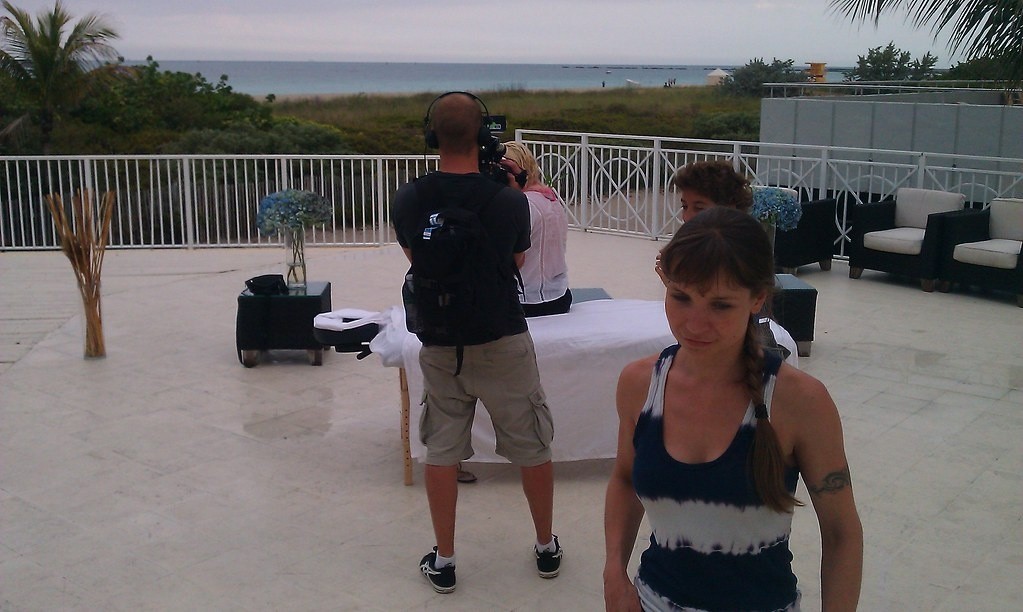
[706,68,729,85]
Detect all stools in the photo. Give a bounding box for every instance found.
[763,272,819,357]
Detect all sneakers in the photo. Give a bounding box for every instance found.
[418,545,458,595]
[533,534,564,578]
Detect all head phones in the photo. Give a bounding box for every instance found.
[424,90,492,149]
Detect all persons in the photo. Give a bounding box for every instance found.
[499,141,573,317]
[664,82,668,88]
[601,80,605,88]
[602,205,864,612]
[655,162,757,288]
[392,91,562,593]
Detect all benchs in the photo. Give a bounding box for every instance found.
[373,305,798,489]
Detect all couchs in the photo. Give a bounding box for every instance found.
[749,185,836,272]
[933,198,1023,309]
[848,188,977,293]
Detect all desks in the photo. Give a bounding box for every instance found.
[235,282,333,366]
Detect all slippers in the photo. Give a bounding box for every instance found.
[457,461,477,481]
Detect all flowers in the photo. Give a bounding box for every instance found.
[256,189,333,236]
[745,187,802,229]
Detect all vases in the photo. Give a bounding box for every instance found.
[284,231,308,290]
[760,222,775,254]
